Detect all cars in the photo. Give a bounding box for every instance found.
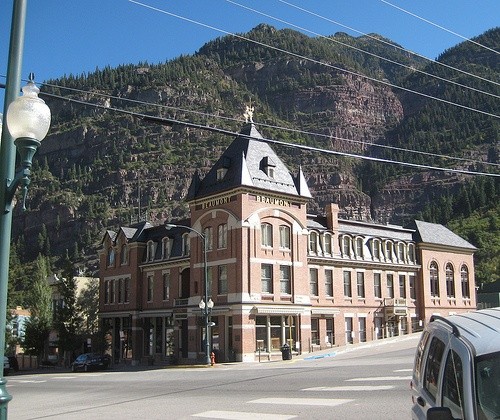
[71,352,110,372]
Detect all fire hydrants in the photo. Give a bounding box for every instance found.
[210,352,215,366]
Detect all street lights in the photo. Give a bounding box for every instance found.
[164,224,215,365]
[0,72,51,420]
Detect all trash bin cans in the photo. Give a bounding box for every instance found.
[279,343,292,360]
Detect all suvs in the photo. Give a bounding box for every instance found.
[411,306,500,420]
